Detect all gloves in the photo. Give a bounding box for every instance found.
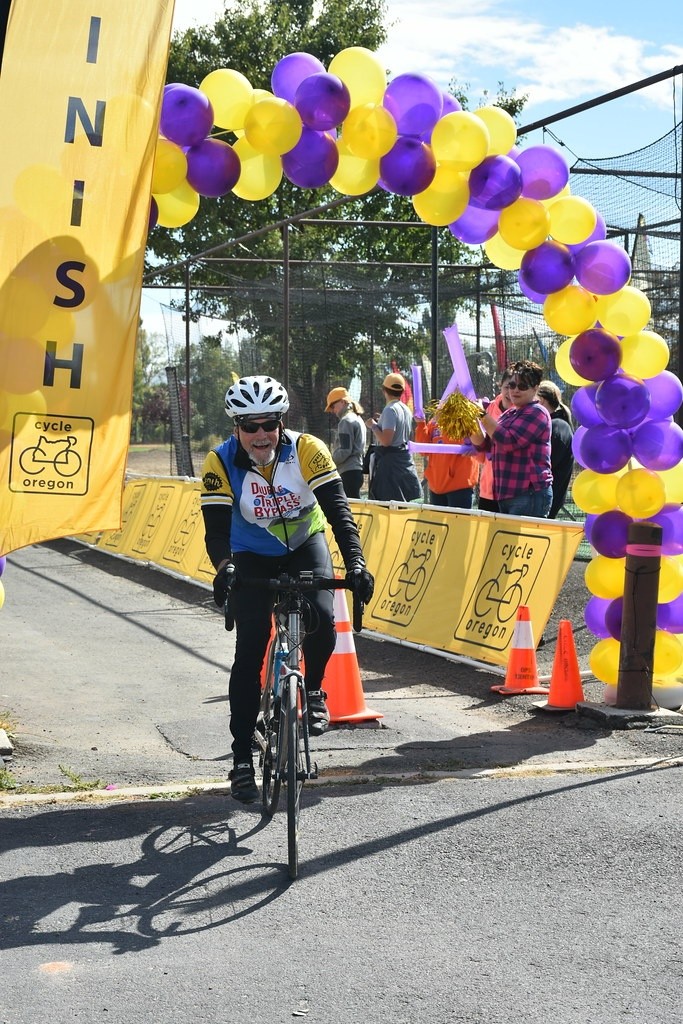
[212,560,236,607]
[345,558,374,605]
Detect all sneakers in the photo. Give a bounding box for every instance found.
[227,770,260,802]
[301,687,330,735]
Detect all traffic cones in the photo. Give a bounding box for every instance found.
[492,605,550,695]
[259,610,310,718]
[319,575,385,724]
[532,619,584,712]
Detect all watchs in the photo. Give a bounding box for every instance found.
[356,557,366,568]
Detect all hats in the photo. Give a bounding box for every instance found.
[324,386,351,412]
[382,373,405,391]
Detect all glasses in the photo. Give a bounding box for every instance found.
[330,399,341,409]
[508,381,535,391]
[235,415,282,433]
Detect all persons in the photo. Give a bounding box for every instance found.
[200,375,374,801]
[325,387,366,499]
[365,373,422,509]
[415,386,485,508]
[477,362,516,510]
[465,360,553,518]
[536,380,574,519]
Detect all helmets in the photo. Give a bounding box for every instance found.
[225,376,290,417]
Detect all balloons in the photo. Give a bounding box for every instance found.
[151,46,683,685]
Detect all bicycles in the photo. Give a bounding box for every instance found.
[224,569,364,879]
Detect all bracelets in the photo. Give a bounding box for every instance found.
[479,411,488,420]
[372,424,378,432]
